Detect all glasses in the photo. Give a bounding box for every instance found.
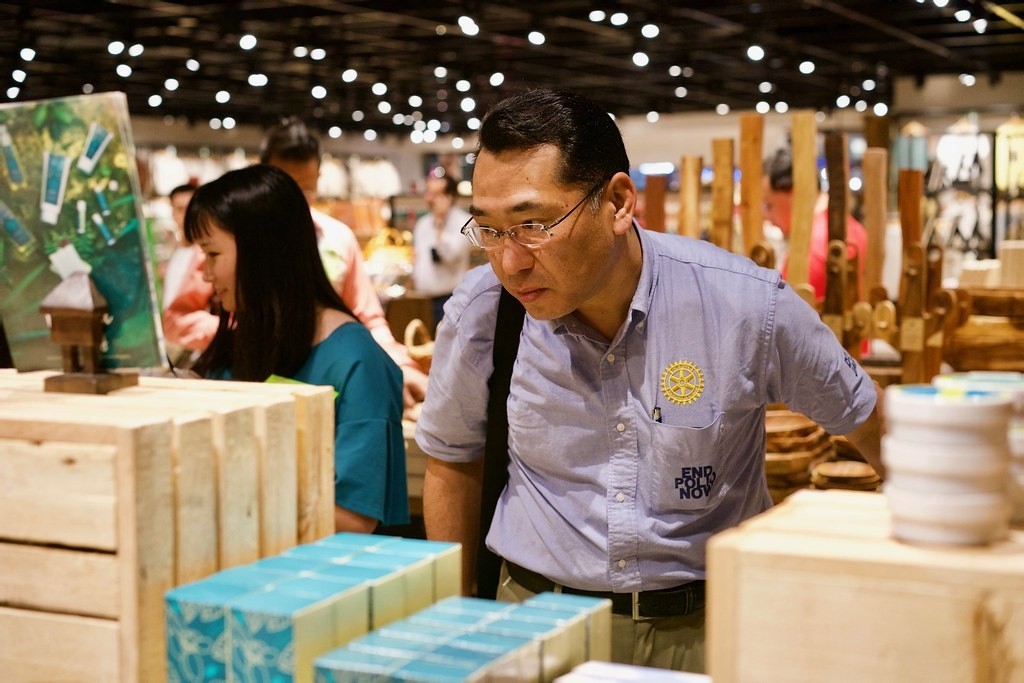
[458,177,609,250]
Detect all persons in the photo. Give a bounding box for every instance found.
[415,171,475,326]
[761,148,867,354]
[162,122,428,408]
[163,184,196,308]
[162,162,410,535]
[319,154,402,227]
[414,88,885,673]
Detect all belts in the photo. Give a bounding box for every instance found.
[504,552,705,622]
